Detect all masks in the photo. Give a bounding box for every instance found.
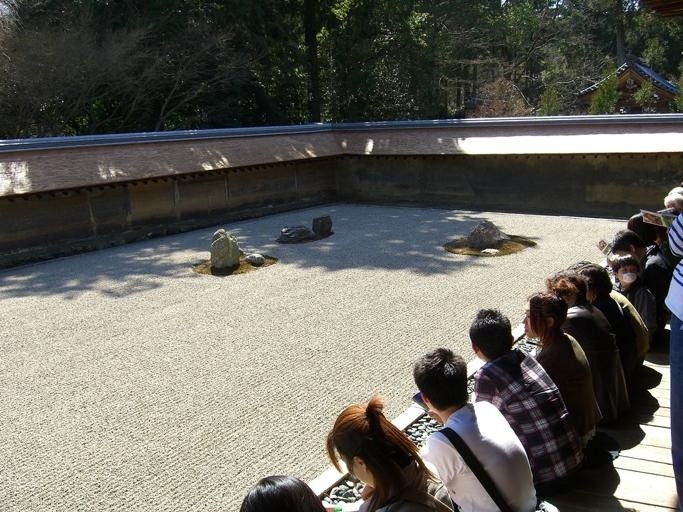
[619,272,637,284]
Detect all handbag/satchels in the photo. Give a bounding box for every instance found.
[533,495,561,512]
